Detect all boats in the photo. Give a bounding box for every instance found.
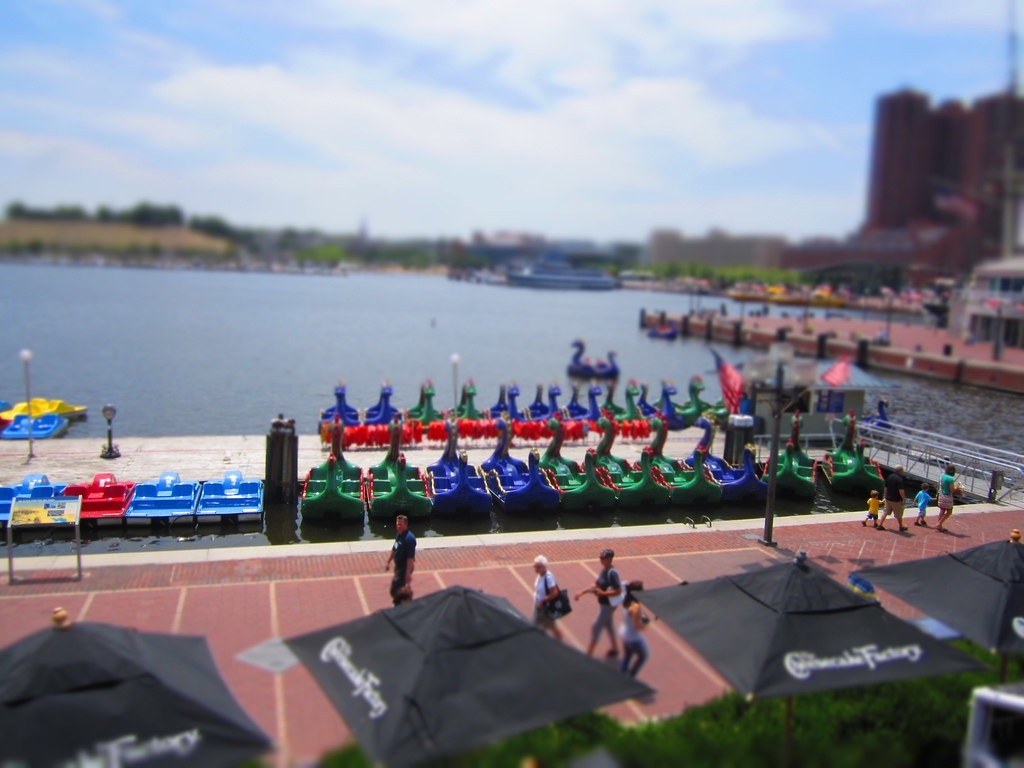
[302,416,888,524]
[726,280,951,314]
[507,258,621,288]
[649,321,680,342]
[565,340,619,380]
[0,474,264,528]
[0,396,87,441]
[317,379,747,427]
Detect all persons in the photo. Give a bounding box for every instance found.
[876,464,909,531]
[533,555,563,641]
[574,548,623,658]
[935,465,960,531]
[741,392,754,415]
[385,515,416,607]
[862,490,885,527]
[913,483,939,526]
[878,396,891,427]
[618,578,650,678]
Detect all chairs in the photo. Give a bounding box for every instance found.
[0,473,264,520]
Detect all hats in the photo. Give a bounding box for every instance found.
[626,582,643,592]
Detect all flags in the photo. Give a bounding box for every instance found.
[712,350,744,415]
[821,361,851,388]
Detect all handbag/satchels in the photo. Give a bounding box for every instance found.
[542,571,572,620]
[605,568,628,607]
[397,583,412,604]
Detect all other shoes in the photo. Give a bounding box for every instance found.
[862,520,866,526]
[914,522,921,526]
[874,523,878,527]
[935,522,946,531]
[899,527,908,531]
[921,520,926,525]
[877,525,885,530]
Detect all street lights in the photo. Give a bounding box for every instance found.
[22,349,36,458]
[760,342,794,545]
[451,353,461,424]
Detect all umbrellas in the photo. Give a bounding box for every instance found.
[632,527,1024,768]
[283,585,658,768]
[0,604,278,768]
